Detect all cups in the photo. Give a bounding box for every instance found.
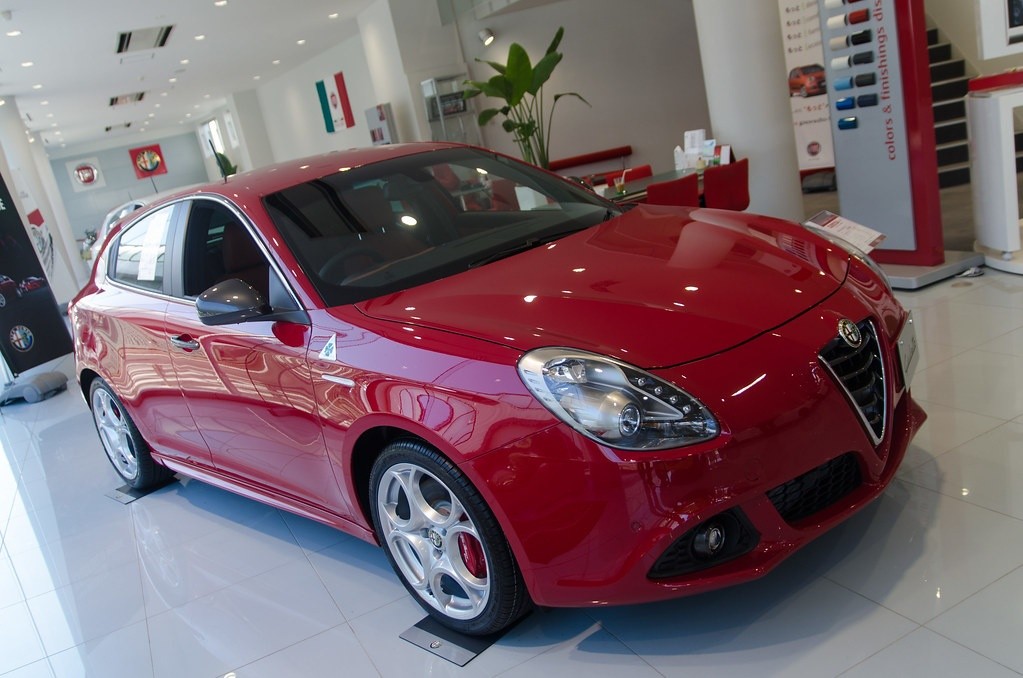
[613,177,625,193]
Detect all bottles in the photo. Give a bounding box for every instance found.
[674,145,685,170]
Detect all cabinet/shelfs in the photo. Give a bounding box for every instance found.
[420,70,484,146]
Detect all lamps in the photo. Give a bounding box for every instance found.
[479,28,494,45]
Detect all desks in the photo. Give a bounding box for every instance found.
[595,168,705,200]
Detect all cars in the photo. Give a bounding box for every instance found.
[16,276,47,299]
[789,63,825,97]
[62,138,929,639]
[0,274,19,309]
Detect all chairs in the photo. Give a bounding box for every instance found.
[646,173,698,208]
[704,158,750,211]
[606,165,652,188]
[214,221,268,294]
[343,186,431,264]
